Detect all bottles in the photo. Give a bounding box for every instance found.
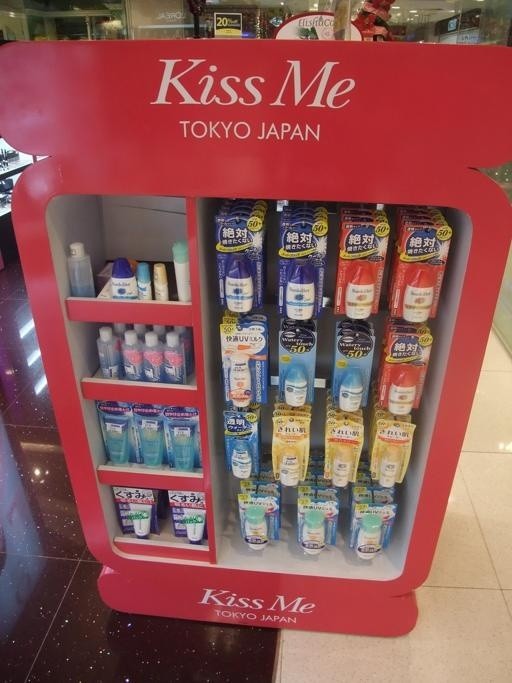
[98,323,186,385]
[63,240,96,300]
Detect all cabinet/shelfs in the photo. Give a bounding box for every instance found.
[1,40,512,637]
[0,136,51,217]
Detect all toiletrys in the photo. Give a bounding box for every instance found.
[225,254,252,313]
[96,240,206,545]
[403,266,434,324]
[67,241,95,296]
[285,264,316,321]
[231,363,420,560]
[346,263,375,320]
